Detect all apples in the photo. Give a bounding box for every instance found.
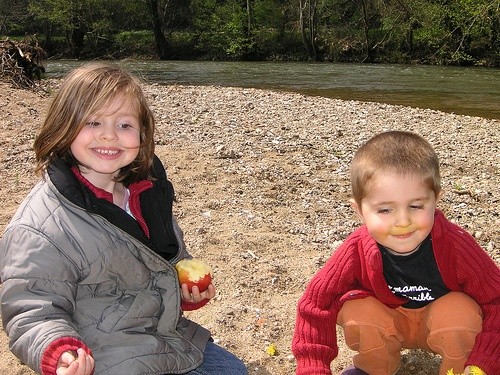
[176,257,215,293]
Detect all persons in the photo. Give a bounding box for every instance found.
[1,60,246,375]
[292,131,500,375]
